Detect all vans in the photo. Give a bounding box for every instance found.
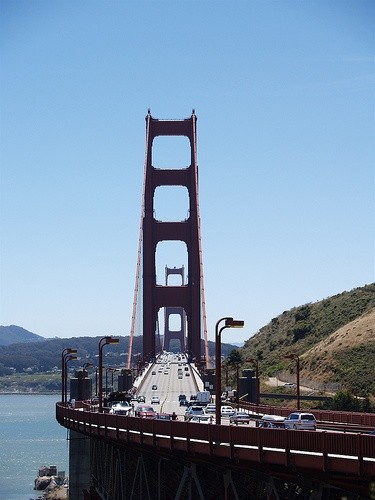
[196,391,212,406]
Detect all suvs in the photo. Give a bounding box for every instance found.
[283,412,317,431]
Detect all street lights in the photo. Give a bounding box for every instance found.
[64,355,78,407]
[230,362,240,409]
[112,368,120,395]
[105,367,115,398]
[285,352,300,410]
[220,364,229,398]
[95,366,105,398]
[246,358,259,413]
[62,347,78,406]
[215,317,245,445]
[82,362,93,401]
[98,336,120,413]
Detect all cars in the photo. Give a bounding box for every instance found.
[259,414,286,429]
[178,395,199,408]
[150,396,161,404]
[206,403,216,413]
[137,396,146,403]
[184,405,210,421]
[229,411,251,424]
[152,350,190,379]
[151,384,158,390]
[220,405,235,417]
[107,398,173,420]
